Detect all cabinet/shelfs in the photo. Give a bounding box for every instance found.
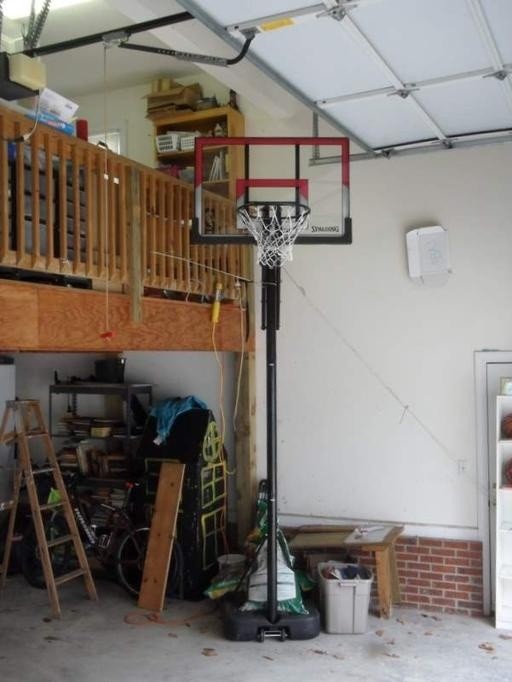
[494,396,512,636]
[152,105,245,201]
[49,378,154,574]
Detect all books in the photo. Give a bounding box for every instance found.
[55,415,127,528]
[208,150,224,182]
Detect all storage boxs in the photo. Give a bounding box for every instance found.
[141,86,202,120]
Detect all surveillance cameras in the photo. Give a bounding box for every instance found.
[101,29,130,45]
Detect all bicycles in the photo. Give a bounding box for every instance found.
[21,472,184,600]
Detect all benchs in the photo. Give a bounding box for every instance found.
[289,525,404,619]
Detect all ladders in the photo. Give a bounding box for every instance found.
[0,400,99,621]
